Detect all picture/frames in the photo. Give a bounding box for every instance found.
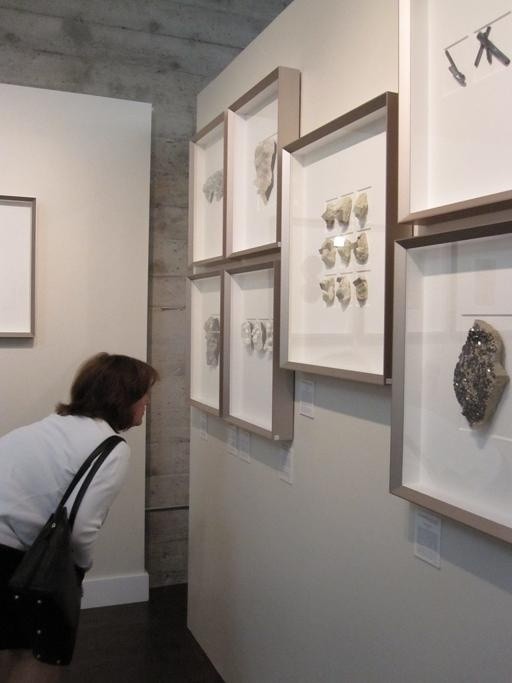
[188,66,301,442]
[0,196,35,340]
[278,90,399,387]
[387,0,511,541]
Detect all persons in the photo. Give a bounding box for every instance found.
[1,351,163,682]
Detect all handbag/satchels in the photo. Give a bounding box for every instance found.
[7,435,127,667]
